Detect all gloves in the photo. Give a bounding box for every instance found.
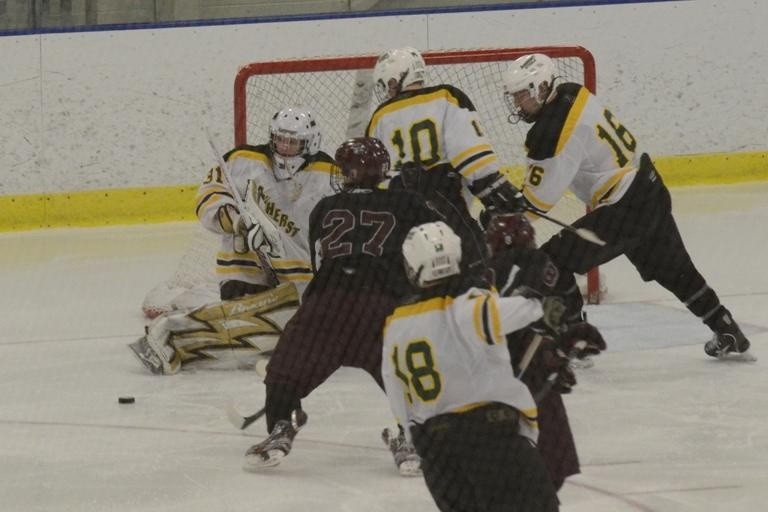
[536,346,577,393]
[531,298,567,340]
[559,323,604,359]
[471,174,527,215]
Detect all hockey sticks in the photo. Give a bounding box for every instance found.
[531,206,606,247]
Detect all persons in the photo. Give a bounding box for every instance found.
[382,221,560,512]
[365,46,520,269]
[478,213,581,493]
[501,53,750,360]
[144,106,343,375]
[242,136,448,467]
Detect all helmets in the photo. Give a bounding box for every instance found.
[402,222,462,289]
[268,109,321,181]
[373,50,427,104]
[332,139,391,191]
[501,55,557,123]
[484,214,537,248]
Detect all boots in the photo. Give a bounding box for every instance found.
[382,429,423,467]
[703,312,750,357]
[246,411,307,457]
